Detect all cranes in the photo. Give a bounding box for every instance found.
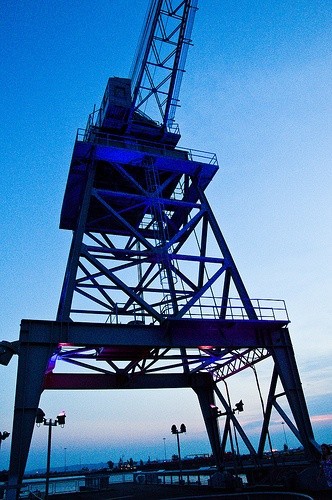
[0,0,327,500]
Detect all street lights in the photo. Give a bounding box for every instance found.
[281,422,288,444]
[64,447,67,473]
[163,438,167,461]
